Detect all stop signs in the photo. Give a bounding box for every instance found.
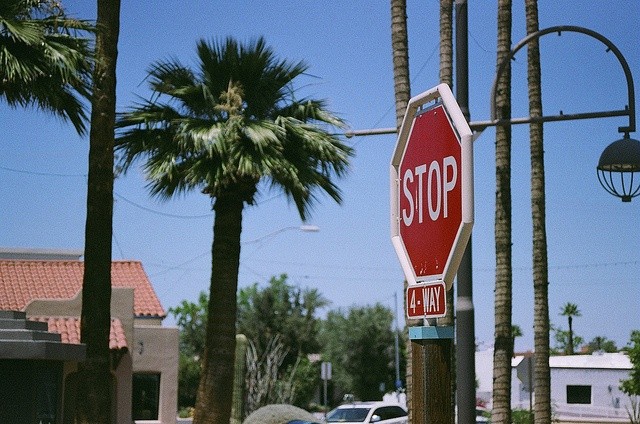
[389,83,474,293]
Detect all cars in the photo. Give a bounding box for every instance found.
[321,400,408,423]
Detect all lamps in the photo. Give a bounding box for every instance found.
[598,129,639,203]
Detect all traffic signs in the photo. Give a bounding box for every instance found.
[405,280,447,320]
